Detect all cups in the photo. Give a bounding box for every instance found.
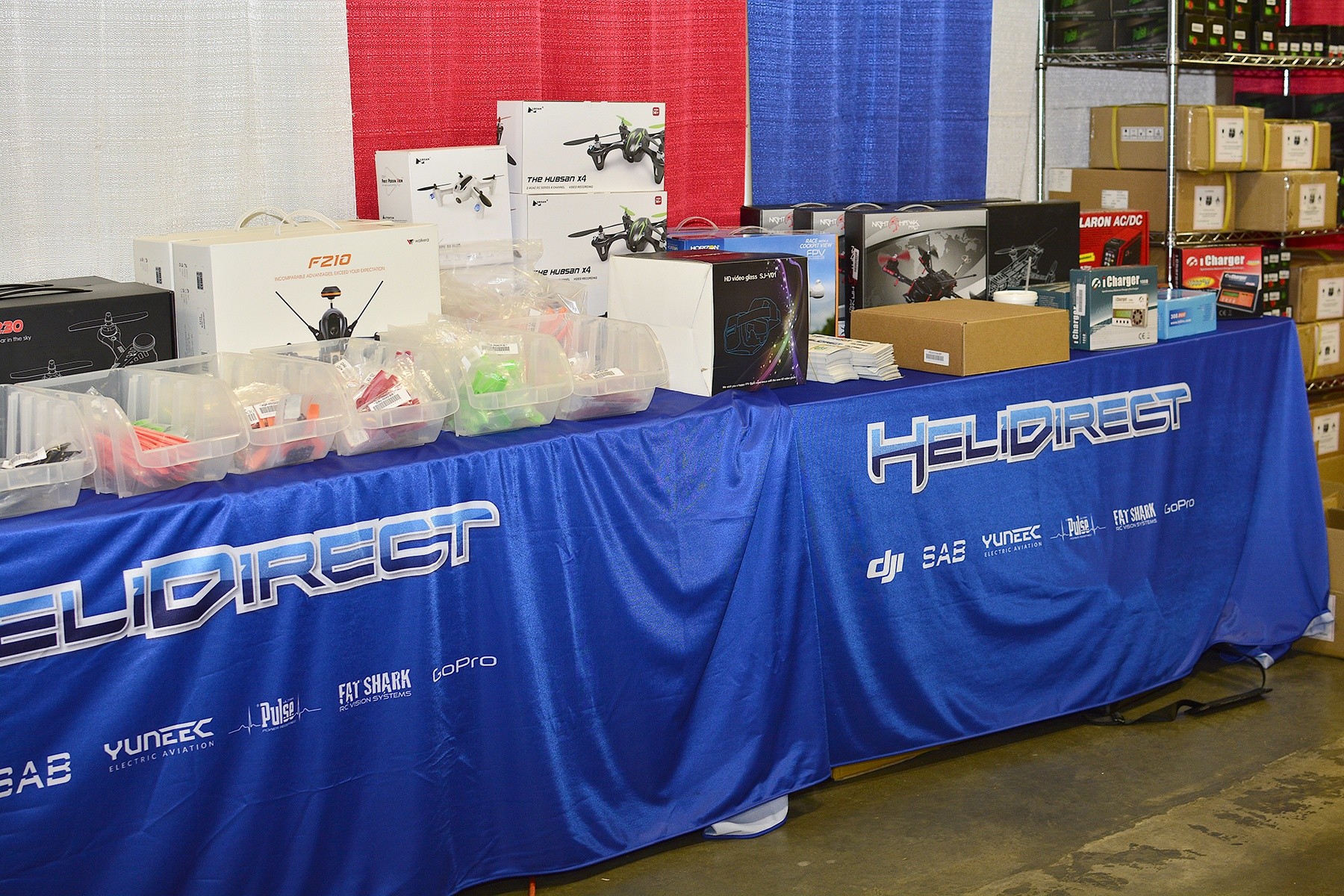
[992,290,1038,307]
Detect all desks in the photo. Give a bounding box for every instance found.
[0,320,1328,896]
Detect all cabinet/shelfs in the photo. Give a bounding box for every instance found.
[1031,0,1343,403]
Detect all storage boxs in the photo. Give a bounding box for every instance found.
[0,0,1344,657]
